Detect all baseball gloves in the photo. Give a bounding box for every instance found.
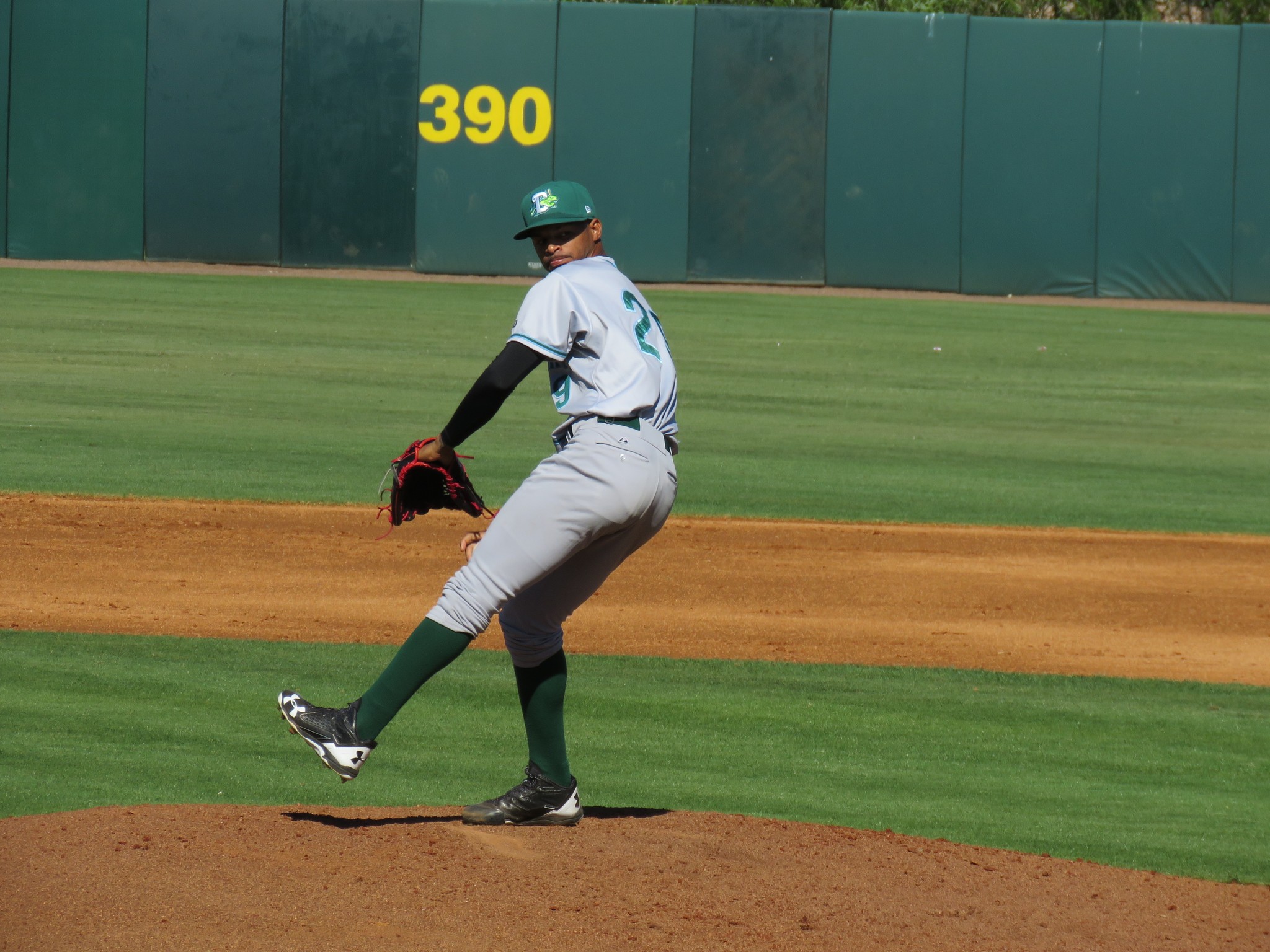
[373,436,500,542]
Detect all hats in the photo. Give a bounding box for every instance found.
[514,181,597,240]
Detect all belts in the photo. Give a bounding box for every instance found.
[597,415,671,455]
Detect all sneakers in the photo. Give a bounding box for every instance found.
[276,690,377,784]
[462,760,584,826]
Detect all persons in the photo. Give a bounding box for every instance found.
[277,178,680,826]
